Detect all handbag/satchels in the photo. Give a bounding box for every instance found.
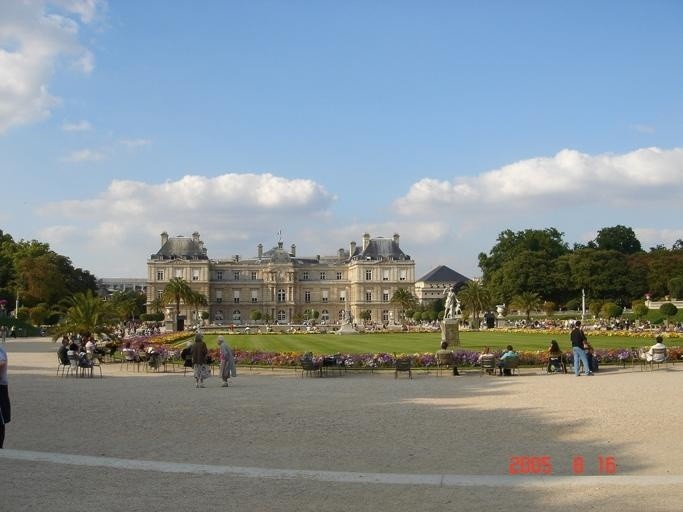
[582,340,589,349]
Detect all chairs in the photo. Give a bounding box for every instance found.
[320,353,343,375]
[392,358,414,381]
[332,353,348,372]
[436,352,459,376]
[477,354,497,376]
[302,356,317,376]
[500,355,518,376]
[649,347,669,371]
[54,340,215,382]
[547,349,566,374]
[637,346,661,372]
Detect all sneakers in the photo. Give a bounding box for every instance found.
[195,383,199,388]
[587,372,594,376]
[220,383,228,387]
[199,384,206,388]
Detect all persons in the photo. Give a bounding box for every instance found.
[0,324,8,344]
[137,344,155,362]
[648,336,669,364]
[9,324,16,339]
[516,315,631,332]
[181,342,194,368]
[0,347,11,450]
[216,334,237,387]
[476,346,497,377]
[582,337,594,373]
[190,334,211,388]
[435,342,462,377]
[442,285,460,319]
[56,333,105,368]
[499,344,521,376]
[545,340,568,374]
[570,320,591,376]
[121,341,142,363]
